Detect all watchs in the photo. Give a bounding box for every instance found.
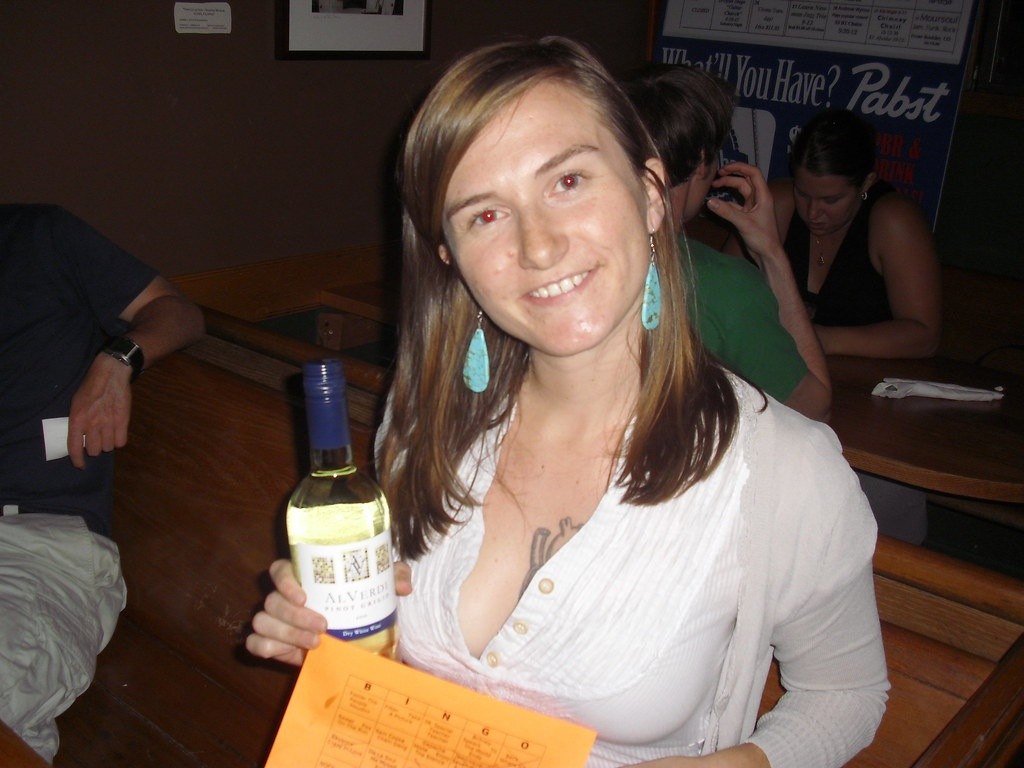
[96,335,144,383]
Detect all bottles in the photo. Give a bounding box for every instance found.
[285,356,399,654]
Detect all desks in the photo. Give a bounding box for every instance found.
[323,288,1024,503]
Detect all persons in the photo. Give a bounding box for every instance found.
[245,34,891,768]
[724,109,943,359]
[619,61,833,423]
[0,203,205,540]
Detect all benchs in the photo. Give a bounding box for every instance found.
[34,297,1024,768]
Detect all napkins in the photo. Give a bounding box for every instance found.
[870,377,1005,402]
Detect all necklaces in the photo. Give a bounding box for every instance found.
[815,236,833,265]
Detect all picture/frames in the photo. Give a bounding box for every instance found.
[273,0,432,61]
